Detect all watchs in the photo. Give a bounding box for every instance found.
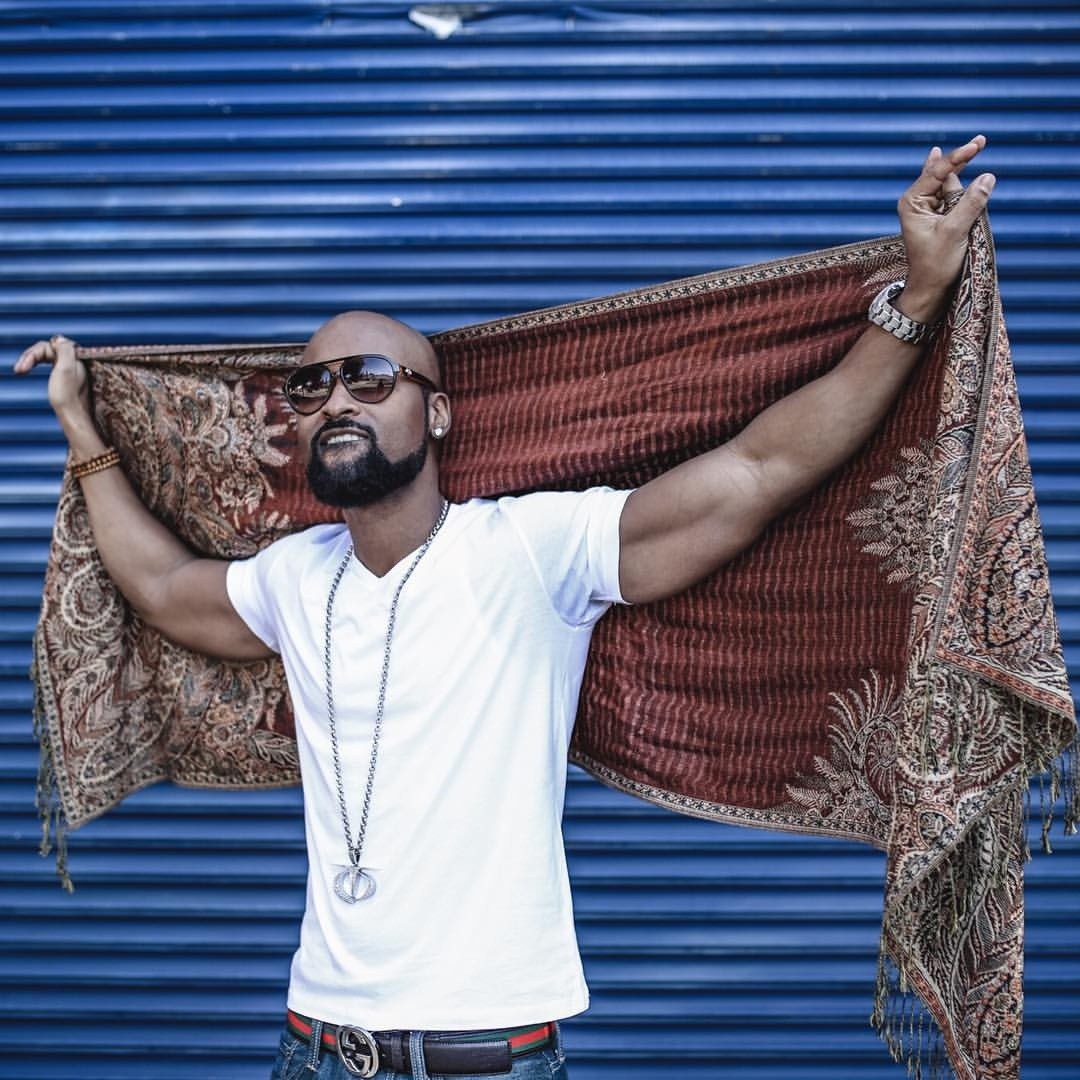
[868,279,942,346]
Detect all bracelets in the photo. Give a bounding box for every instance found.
[68,445,121,479]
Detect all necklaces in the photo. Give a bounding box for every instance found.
[324,499,450,905]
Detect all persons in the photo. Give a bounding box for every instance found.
[15,134,996,1080]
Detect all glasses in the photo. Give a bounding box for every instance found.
[282,353,441,417]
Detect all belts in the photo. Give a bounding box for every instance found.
[285,1004,553,1077]
